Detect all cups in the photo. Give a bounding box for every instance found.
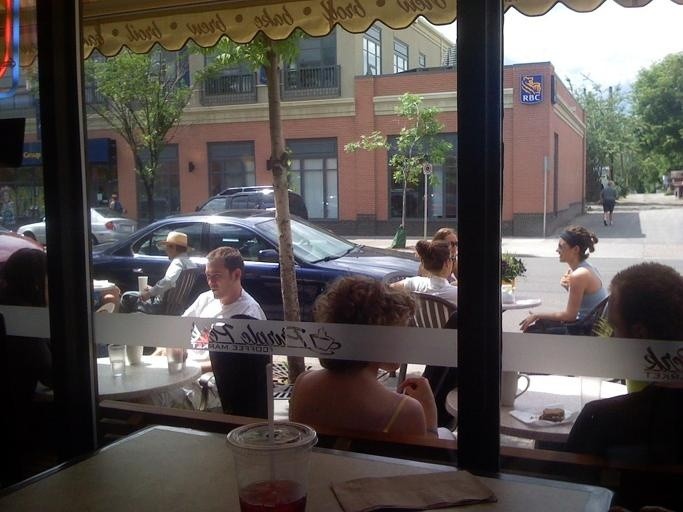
[500,370,529,407]
[138,275,149,294]
[226,419,319,512]
[501,284,515,305]
[104,340,184,376]
[580,375,602,409]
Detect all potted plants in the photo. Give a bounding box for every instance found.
[501,252,527,302]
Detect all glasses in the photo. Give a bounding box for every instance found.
[448,255,456,263]
[447,241,458,249]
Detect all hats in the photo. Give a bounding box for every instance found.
[159,230,196,254]
[111,194,119,198]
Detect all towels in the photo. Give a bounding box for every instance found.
[328,469,498,511]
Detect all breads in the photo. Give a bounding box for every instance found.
[543,408,565,422]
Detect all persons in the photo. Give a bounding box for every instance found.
[122,246,269,433]
[108,193,122,216]
[517,224,609,334]
[283,270,439,465]
[388,238,458,328]
[563,259,683,512]
[418,226,458,286]
[117,230,198,315]
[599,180,619,227]
[0,185,17,228]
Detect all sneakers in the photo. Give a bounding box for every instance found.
[603,219,613,226]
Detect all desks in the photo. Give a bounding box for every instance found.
[443,373,629,452]
[96,354,202,399]
[501,298,543,317]
[0,421,614,512]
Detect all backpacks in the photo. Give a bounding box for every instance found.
[113,199,123,214]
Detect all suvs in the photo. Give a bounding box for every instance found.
[193,183,312,222]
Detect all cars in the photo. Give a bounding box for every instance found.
[17,204,138,246]
[92,209,423,325]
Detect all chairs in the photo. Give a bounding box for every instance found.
[394,289,456,393]
[94,302,115,316]
[197,364,216,412]
[379,268,421,293]
[120,263,205,312]
[522,292,611,336]
